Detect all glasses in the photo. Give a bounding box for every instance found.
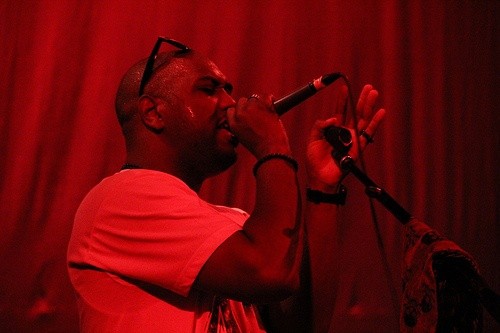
[138,35,191,97]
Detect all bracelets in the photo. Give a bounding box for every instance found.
[253,154,298,174]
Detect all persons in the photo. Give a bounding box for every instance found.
[67,36,385,333]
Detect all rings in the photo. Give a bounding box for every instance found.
[247,94,260,102]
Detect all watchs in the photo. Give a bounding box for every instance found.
[306,184,348,206]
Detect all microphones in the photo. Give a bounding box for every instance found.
[273,72,343,116]
